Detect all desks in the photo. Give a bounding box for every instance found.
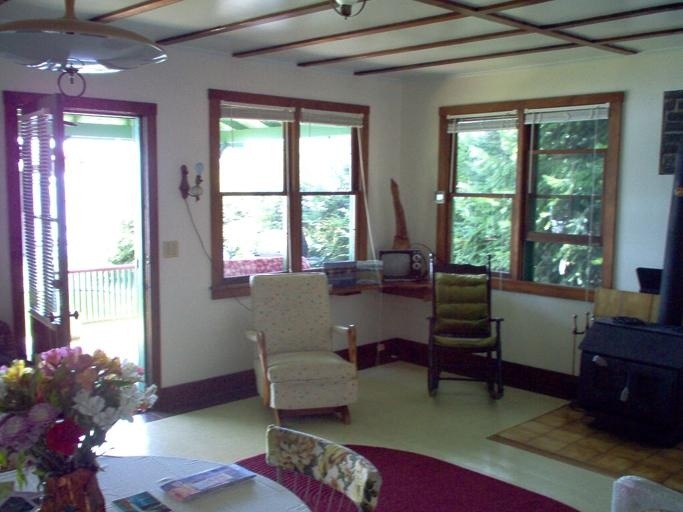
[1,456,311,512]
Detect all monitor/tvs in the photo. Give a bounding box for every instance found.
[378,248,424,281]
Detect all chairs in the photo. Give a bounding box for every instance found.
[265,423,381,512]
[425,261,504,400]
[245,273,357,426]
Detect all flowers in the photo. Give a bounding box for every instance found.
[1,346,157,488]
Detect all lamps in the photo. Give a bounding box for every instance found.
[0,0,168,97]
[178,162,204,202]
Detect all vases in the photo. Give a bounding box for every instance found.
[40,463,106,512]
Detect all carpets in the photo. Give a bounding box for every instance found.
[234,444,589,512]
